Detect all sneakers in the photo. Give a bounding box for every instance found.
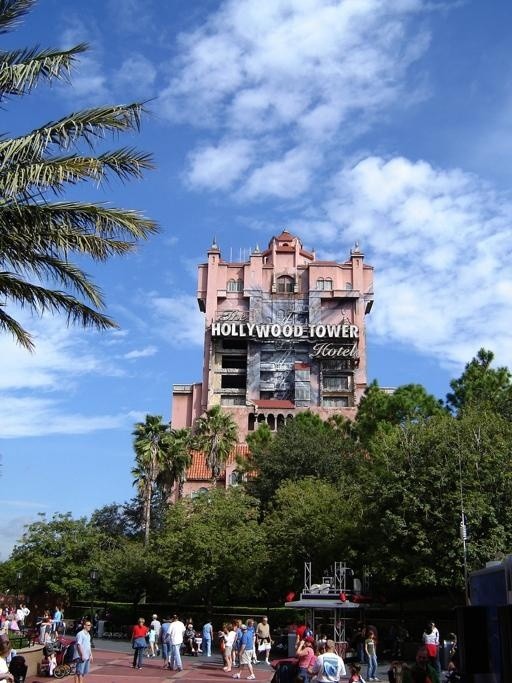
[130,648,183,672]
[192,648,212,657]
[222,659,271,680]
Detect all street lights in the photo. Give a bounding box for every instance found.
[87,564,98,648]
[16,568,24,605]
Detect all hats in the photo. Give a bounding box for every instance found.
[0,641,13,654]
[304,636,313,643]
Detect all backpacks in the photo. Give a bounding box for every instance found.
[303,627,314,637]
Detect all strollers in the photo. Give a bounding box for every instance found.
[26,616,77,679]
[271,658,312,682]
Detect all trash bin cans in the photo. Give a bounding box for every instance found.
[98,620,109,640]
[288,630,297,658]
